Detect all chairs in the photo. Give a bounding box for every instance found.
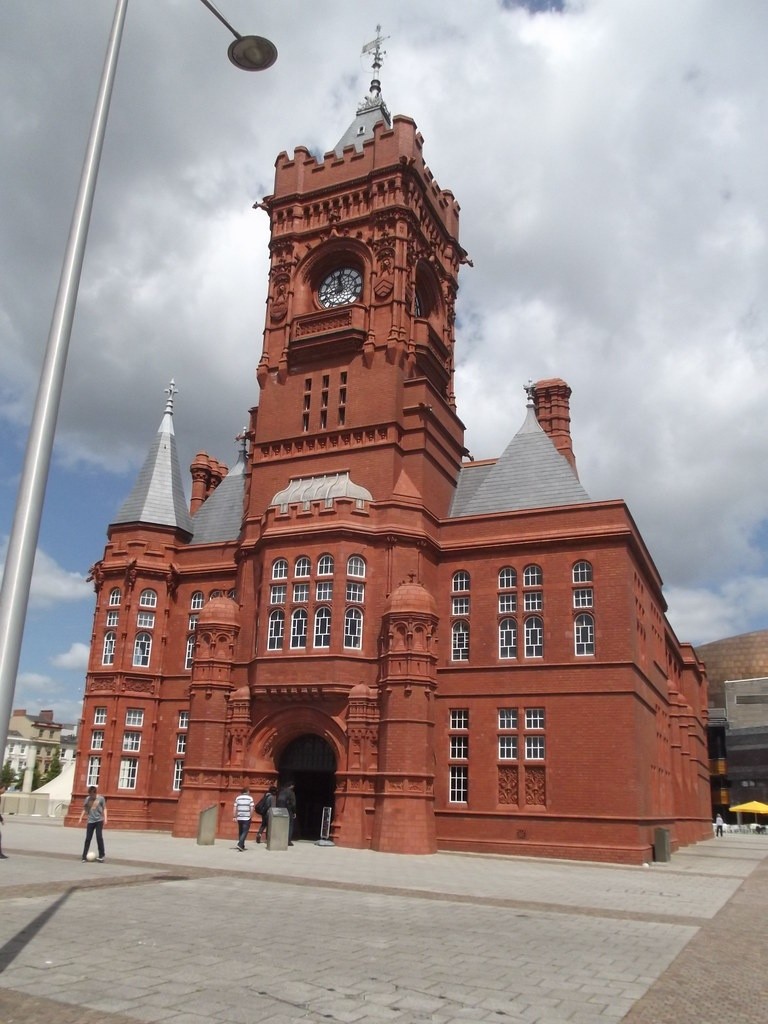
[712,822,768,835]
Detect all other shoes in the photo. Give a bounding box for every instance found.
[81,859,86,863]
[0,854,9,859]
[255,835,261,843]
[236,843,244,851]
[288,842,293,846]
[96,856,103,861]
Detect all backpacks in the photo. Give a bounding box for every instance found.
[255,792,271,815]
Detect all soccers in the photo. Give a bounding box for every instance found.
[86,852,96,862]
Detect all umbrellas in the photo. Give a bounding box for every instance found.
[728,800,768,824]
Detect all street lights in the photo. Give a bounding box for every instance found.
[0,0,279,768]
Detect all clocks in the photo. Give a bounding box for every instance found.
[317,265,364,309]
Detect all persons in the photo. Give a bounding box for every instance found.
[256,786,278,843]
[232,787,255,852]
[77,786,107,862]
[715,813,724,837]
[278,781,297,846]
[0,781,8,860]
